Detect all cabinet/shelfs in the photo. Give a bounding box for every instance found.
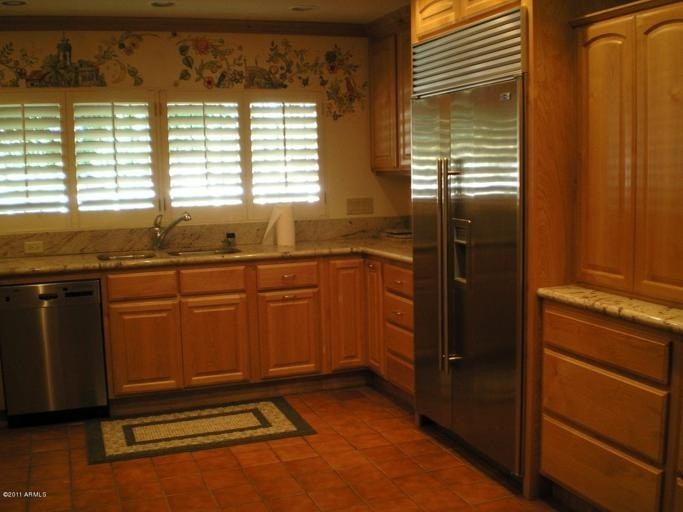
[537,296,682,512]
[327,254,365,374]
[99,261,252,400]
[254,258,322,385]
[566,1,682,309]
[364,257,382,381]
[409,1,526,48]
[367,22,411,174]
[381,261,416,399]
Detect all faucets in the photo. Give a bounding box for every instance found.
[156,211,191,242]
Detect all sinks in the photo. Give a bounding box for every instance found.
[96,252,158,260]
[168,247,241,256]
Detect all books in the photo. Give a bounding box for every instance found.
[383,228,412,238]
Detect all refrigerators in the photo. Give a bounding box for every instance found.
[409,73,525,487]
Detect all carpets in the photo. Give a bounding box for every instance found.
[83,395,319,466]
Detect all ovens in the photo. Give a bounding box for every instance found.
[0,279,111,428]
